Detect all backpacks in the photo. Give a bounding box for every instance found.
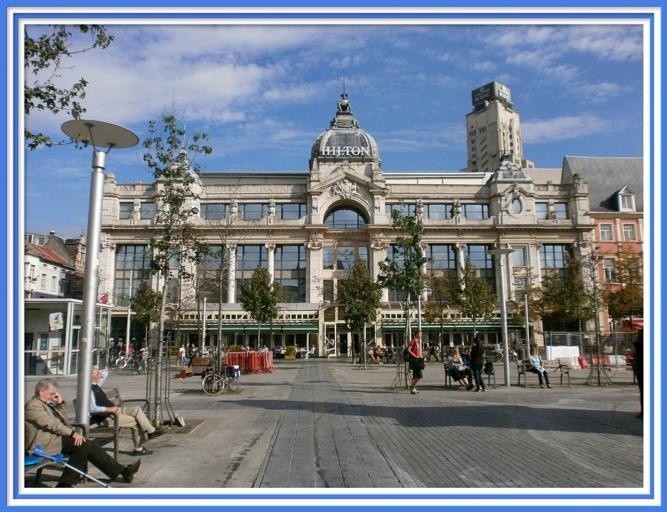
[404,347,413,362]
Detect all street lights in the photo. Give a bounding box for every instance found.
[486,247,515,386]
[198,290,212,357]
[61,119,138,440]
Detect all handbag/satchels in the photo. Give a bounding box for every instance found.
[414,357,424,370]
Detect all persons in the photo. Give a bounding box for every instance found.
[25,378,141,488]
[263,343,316,359]
[632,329,643,418]
[90,367,169,456]
[129,343,198,368]
[367,343,398,364]
[333,179,359,199]
[408,330,552,394]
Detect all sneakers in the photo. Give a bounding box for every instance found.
[410,385,417,394]
[540,383,552,389]
[464,383,486,392]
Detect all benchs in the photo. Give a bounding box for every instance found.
[25,387,151,489]
[516,359,572,388]
[444,362,495,388]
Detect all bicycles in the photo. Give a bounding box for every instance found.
[202,364,240,396]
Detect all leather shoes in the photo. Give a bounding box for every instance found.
[148,430,164,440]
[123,457,141,483]
[133,446,153,456]
